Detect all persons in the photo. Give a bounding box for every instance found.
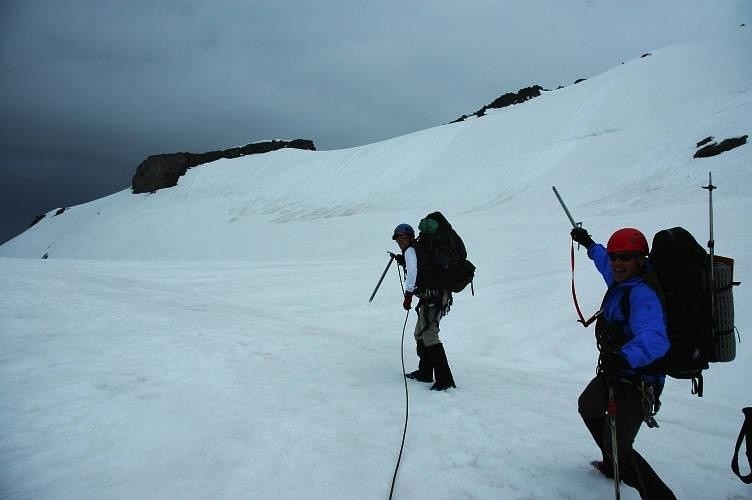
[568,225,678,500]
[392,223,457,392]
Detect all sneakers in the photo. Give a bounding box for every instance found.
[590,460,623,482]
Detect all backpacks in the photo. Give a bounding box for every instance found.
[594,226,711,398]
[402,211,475,298]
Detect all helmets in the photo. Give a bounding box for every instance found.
[606,227,650,255]
[392,223,415,240]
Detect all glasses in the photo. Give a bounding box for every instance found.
[609,253,637,262]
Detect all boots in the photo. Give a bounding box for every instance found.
[428,345,457,390]
[405,344,434,383]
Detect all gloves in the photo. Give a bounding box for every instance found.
[569,227,594,248]
[402,294,413,310]
[603,351,633,372]
